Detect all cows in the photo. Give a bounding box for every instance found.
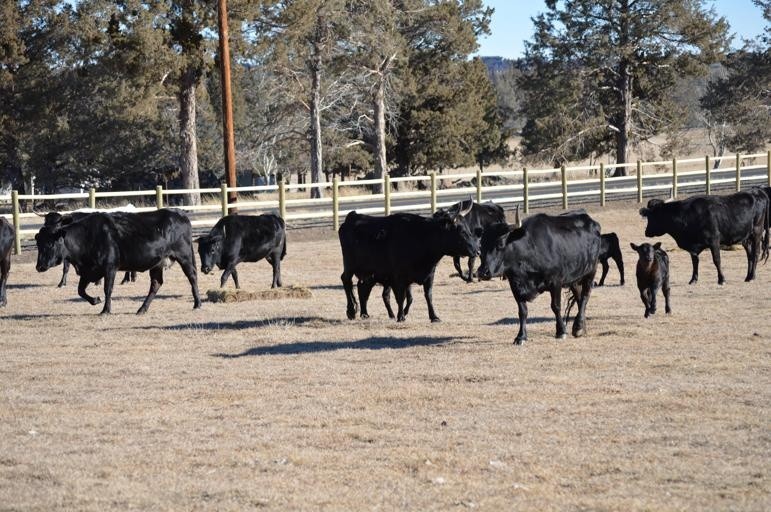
[24,204,202,317]
[0,216,16,309]
[638,184,771,286]
[477,208,609,346]
[337,193,481,324]
[629,241,672,318]
[598,232,625,287]
[447,198,505,284]
[192,211,286,289]
